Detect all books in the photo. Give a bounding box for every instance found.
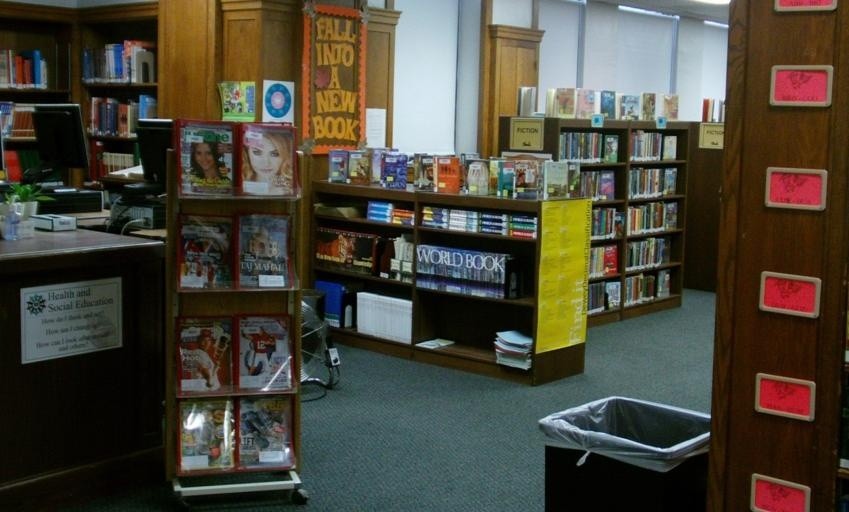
[1,39,158,181]
[181,124,294,472]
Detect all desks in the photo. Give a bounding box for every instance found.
[0,223,171,512]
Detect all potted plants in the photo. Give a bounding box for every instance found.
[4,180,56,223]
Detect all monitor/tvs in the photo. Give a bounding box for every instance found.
[121,118,176,200]
[23,103,90,188]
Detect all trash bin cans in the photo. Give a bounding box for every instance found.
[538,394,711,512]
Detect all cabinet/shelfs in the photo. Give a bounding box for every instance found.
[164,146,302,502]
[0,1,76,189]
[620,122,691,321]
[306,176,415,360]
[498,117,628,327]
[414,190,593,386]
[77,5,159,194]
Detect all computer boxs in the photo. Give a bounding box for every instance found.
[112,199,166,229]
[31,190,104,214]
